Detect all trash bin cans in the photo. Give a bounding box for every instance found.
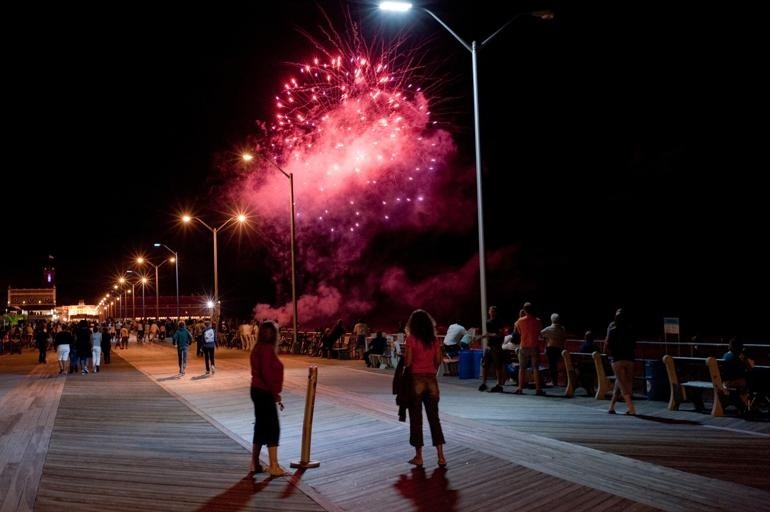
[645,360,670,401]
[458,350,483,379]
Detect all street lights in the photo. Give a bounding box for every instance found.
[377,1,554,355]
[153,243,181,322]
[95,275,135,324]
[136,256,176,322]
[126,270,145,320]
[180,209,245,337]
[241,149,298,344]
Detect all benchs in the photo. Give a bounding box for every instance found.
[279,327,407,356]
[561,350,616,400]
[661,355,763,415]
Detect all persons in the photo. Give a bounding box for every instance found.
[395,309,448,468]
[278,318,407,368]
[1,318,262,376]
[442,303,768,416]
[249,320,295,478]
